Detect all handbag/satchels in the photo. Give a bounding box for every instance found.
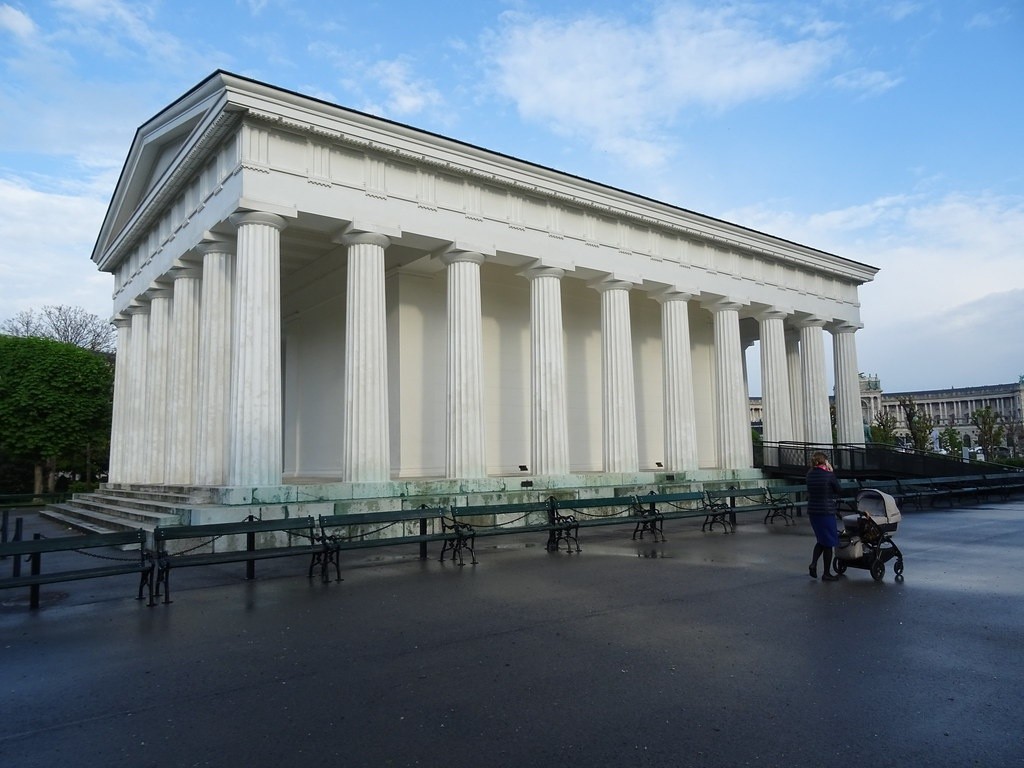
[834,537,863,560]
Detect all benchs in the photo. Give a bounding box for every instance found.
[861,480,920,514]
[898,478,952,511]
[837,483,862,512]
[317,505,468,583]
[704,487,791,533]
[767,484,842,526]
[551,495,660,552]
[0,527,159,609]
[153,515,334,605]
[636,490,729,542]
[446,498,574,564]
[931,472,1024,506]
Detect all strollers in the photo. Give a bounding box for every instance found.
[832,488,905,581]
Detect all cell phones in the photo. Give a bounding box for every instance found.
[826,461,829,468]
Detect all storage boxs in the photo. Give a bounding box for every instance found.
[835,535,864,559]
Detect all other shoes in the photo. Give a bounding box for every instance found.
[809,564,818,578]
[822,574,839,582]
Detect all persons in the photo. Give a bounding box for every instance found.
[806,451,844,581]
